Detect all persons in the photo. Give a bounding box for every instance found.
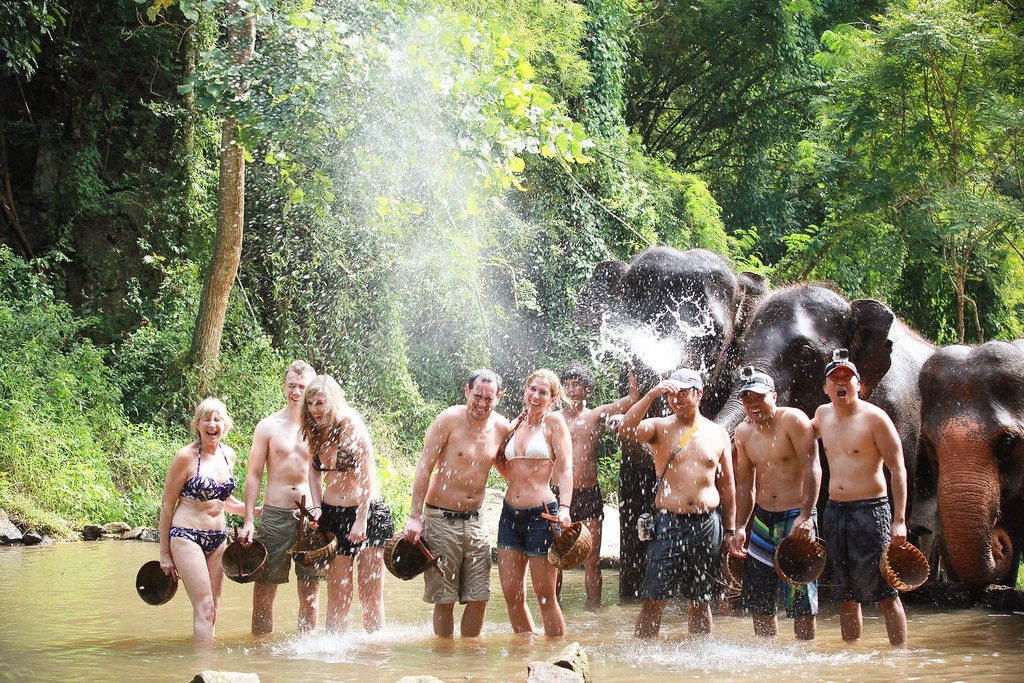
[298,374,394,633]
[238,360,318,637]
[404,369,510,638]
[809,361,908,644]
[496,367,574,636]
[618,369,736,635]
[549,363,640,601]
[159,397,263,638]
[729,374,822,639]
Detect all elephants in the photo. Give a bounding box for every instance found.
[576,246,769,605]
[918,339,1024,590]
[712,285,941,582]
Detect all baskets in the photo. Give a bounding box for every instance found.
[879,540,931,592]
[701,547,747,599]
[288,494,339,568]
[221,523,269,584]
[773,535,828,586]
[136,560,181,607]
[540,501,593,572]
[383,530,445,581]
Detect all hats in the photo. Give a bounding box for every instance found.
[739,373,775,395]
[823,360,860,382]
[667,368,705,390]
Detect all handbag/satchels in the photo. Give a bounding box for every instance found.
[636,513,655,542]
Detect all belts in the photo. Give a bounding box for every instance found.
[444,512,478,521]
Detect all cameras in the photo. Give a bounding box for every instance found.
[636,512,656,541]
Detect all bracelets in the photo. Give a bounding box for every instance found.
[559,504,571,508]
[724,529,735,534]
[314,507,321,509]
